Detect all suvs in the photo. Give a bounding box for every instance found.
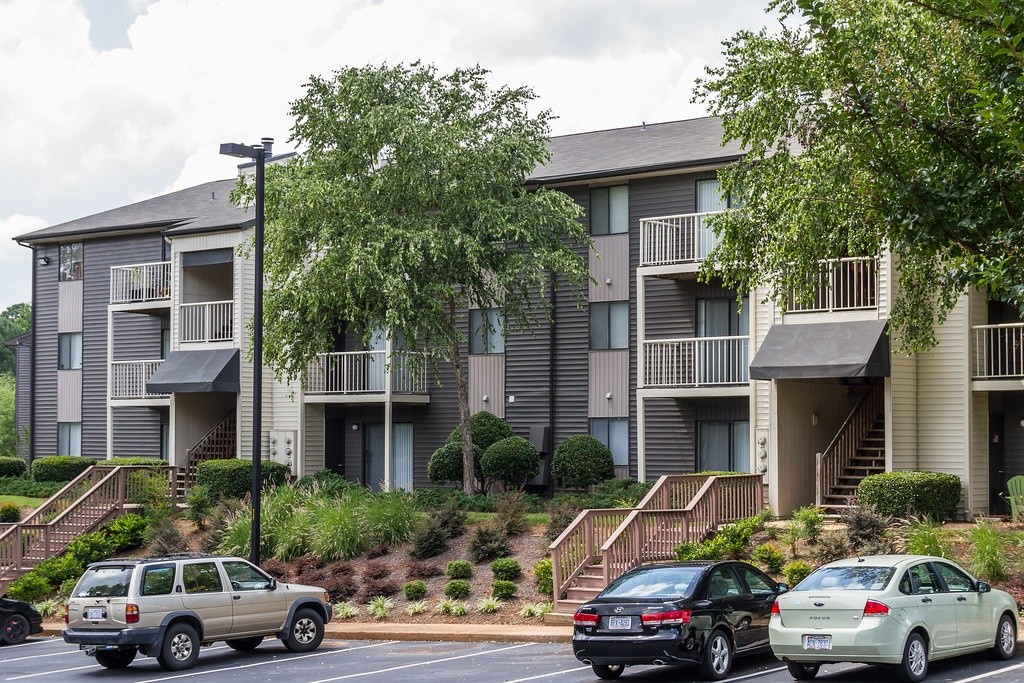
[62,552,333,672]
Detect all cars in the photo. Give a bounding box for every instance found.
[0,596,43,646]
[768,553,1020,683]
[571,560,789,682]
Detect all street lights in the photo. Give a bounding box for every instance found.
[220,143,267,567]
